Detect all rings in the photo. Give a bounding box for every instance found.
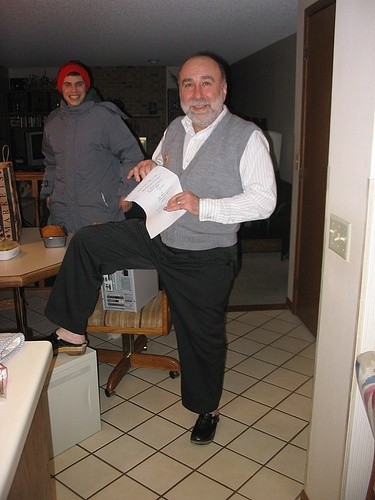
[177,200,180,205]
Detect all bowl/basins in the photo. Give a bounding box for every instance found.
[0,241,21,260]
[39,226,68,248]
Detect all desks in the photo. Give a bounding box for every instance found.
[0,227,75,341]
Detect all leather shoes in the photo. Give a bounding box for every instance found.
[190,410,220,446]
[28,333,88,354]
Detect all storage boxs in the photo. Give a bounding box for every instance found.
[47,344,102,456]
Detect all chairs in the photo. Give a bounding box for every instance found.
[86,280,180,397]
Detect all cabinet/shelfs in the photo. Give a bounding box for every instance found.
[0,336,57,500]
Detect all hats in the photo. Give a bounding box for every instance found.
[56,60,90,94]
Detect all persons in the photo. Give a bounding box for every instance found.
[40,64,144,233]
[44,57,278,445]
